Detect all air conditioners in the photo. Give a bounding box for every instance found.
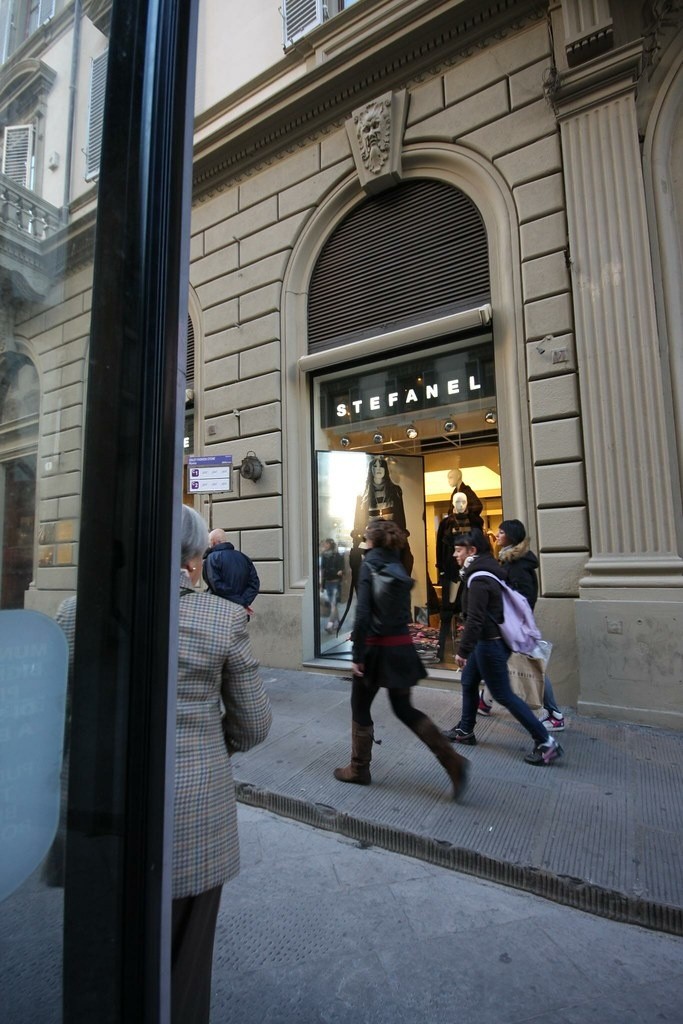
[280,0,339,52]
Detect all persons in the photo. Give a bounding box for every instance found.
[349,531,358,600]
[478,519,564,731]
[441,530,566,766]
[320,540,344,634]
[202,527,260,623]
[447,468,483,515]
[435,491,487,663]
[353,457,411,554]
[334,521,472,800]
[44,503,272,1024]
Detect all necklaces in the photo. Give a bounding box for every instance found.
[373,486,386,518]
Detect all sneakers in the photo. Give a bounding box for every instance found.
[476,689,492,716]
[440,721,477,745]
[524,736,564,766]
[538,706,565,732]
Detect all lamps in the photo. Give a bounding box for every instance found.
[444,415,457,431]
[372,428,384,445]
[340,432,352,448]
[485,408,497,424]
[406,421,419,439]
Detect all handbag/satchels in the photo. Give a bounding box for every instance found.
[320,588,329,602]
[528,639,553,671]
[504,650,545,710]
[364,561,415,639]
[467,571,541,654]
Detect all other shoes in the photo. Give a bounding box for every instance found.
[325,623,334,634]
[333,620,339,630]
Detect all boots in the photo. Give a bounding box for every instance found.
[412,716,470,800]
[334,721,381,786]
